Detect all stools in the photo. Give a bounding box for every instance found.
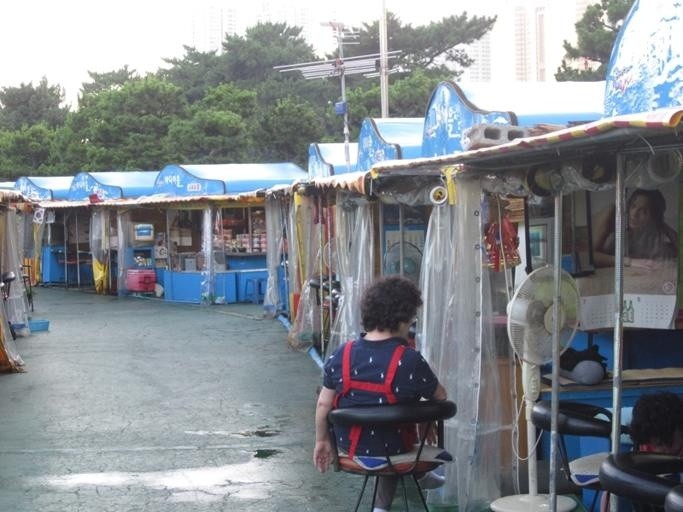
[244,277,267,304]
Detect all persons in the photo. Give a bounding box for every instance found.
[600,389,683,512]
[592,188,678,270]
[310,271,449,512]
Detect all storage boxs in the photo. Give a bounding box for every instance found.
[128,269,156,292]
[29,320,49,331]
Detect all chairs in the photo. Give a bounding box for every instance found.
[599,451,683,512]
[310,274,342,354]
[50,248,117,289]
[531,399,632,512]
[0,265,34,317]
[327,400,457,512]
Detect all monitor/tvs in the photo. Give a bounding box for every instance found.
[527,218,554,265]
[135,224,154,241]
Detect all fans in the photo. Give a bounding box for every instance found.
[383,240,423,290]
[323,237,339,274]
[489,264,581,512]
